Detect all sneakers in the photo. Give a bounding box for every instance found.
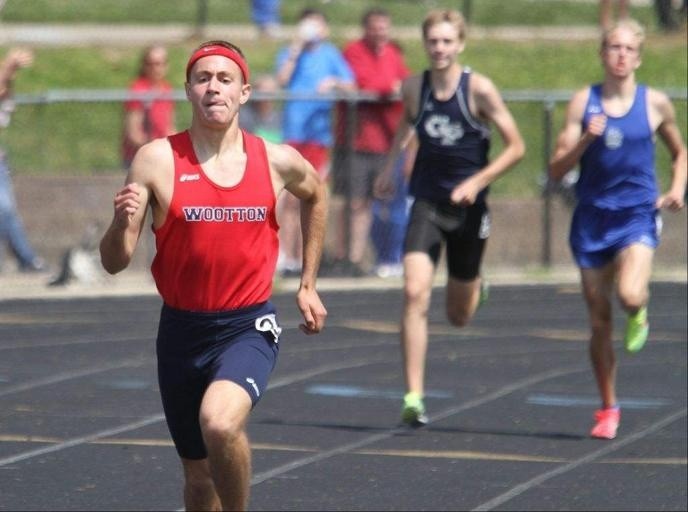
[624,308,650,353]
[401,393,429,426]
[591,406,622,440]
[480,282,488,304]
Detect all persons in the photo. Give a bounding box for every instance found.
[373,10,526,425]
[1,45,48,270]
[120,44,173,169]
[100,41,330,512]
[239,1,418,280]
[550,0,688,439]
